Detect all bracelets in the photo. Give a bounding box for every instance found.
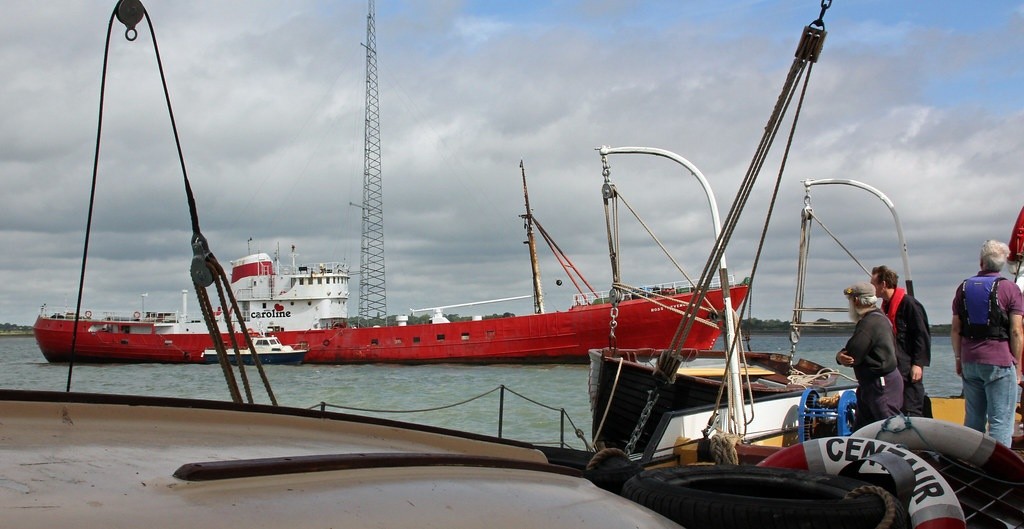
[955,356,961,361]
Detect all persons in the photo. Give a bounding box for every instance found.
[871,264,931,418]
[835,283,902,432]
[948,238,1023,450]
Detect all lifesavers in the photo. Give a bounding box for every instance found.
[134,311,140,318]
[85,310,92,318]
[850,416,1024,485]
[622,462,913,529]
[755,435,966,529]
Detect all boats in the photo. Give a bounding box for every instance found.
[35,235,751,364]
[0,389,1024,529]
[927,393,1022,426]
[204,336,307,365]
[586,144,840,457]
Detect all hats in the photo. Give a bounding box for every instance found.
[843,283,877,298]
